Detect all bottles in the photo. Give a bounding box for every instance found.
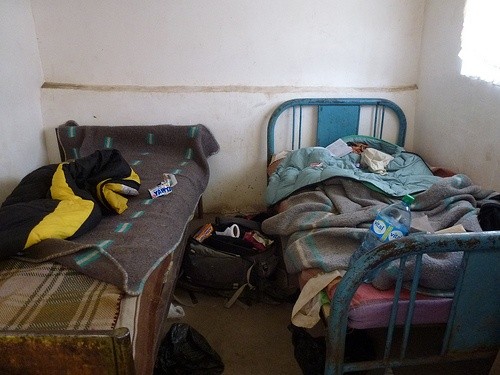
[348,195,415,284]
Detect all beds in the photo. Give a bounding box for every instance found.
[261,98,500,375]
[0,120,219,375]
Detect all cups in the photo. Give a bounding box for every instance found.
[215,224,240,238]
